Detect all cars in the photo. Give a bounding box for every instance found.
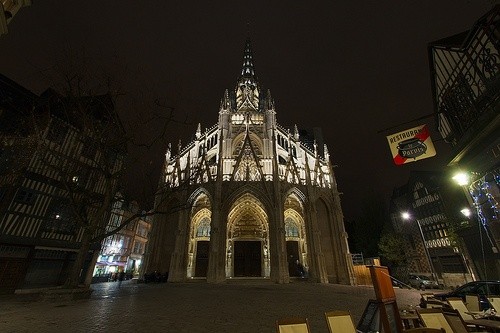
[406,274,438,288]
[420,280,500,311]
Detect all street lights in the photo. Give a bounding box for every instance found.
[402,213,439,283]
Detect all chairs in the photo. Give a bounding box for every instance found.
[441,309,469,333]
[274,316,310,332]
[445,296,478,327]
[419,292,436,301]
[325,308,355,333]
[405,326,446,333]
[425,301,448,314]
[417,307,457,332]
[427,296,442,301]
[465,293,479,312]
[483,292,500,314]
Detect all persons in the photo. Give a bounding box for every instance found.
[95,266,134,289]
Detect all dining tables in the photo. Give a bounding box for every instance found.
[396,302,421,326]
[462,315,500,333]
